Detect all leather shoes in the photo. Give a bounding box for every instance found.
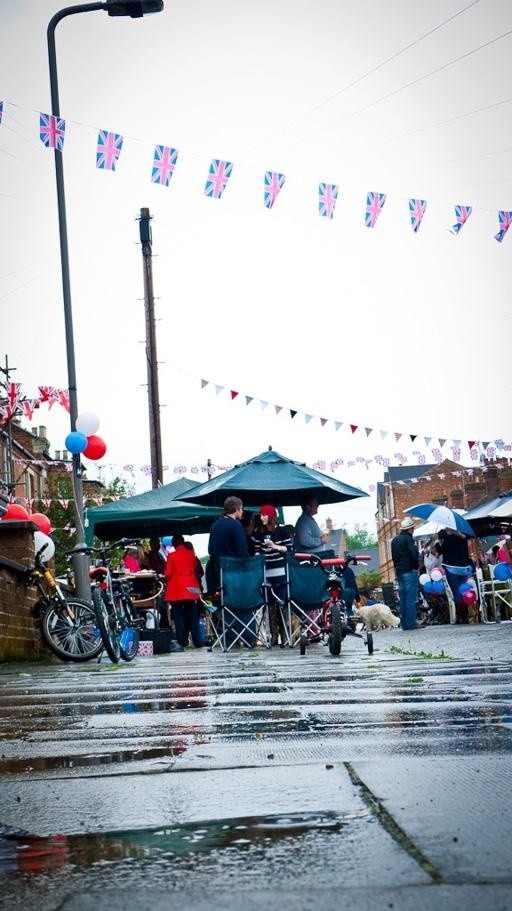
[414,625,426,628]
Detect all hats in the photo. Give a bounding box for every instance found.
[400,518,415,530]
[260,505,276,519]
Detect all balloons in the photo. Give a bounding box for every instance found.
[65,432,88,454]
[65,412,107,460]
[75,411,100,437]
[83,435,106,460]
[1,502,56,564]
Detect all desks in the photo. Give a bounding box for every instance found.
[480,579,512,624]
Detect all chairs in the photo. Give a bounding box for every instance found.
[286,560,326,646]
[219,554,272,651]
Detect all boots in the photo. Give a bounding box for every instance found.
[267,605,278,646]
[278,603,289,647]
[453,603,464,624]
[463,604,469,624]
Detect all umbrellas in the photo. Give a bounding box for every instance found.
[170,445,370,508]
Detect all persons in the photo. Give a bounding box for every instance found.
[391,518,426,630]
[206,496,362,647]
[123,535,205,647]
[417,527,510,625]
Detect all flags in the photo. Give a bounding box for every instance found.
[0,379,72,425]
[203,159,234,199]
[364,191,387,228]
[319,182,340,220]
[39,112,66,153]
[149,143,179,187]
[96,129,124,172]
[264,171,286,209]
[409,199,427,233]
[2,494,121,512]
[493,210,512,244]
[447,205,472,236]
[0,100,4,123]
